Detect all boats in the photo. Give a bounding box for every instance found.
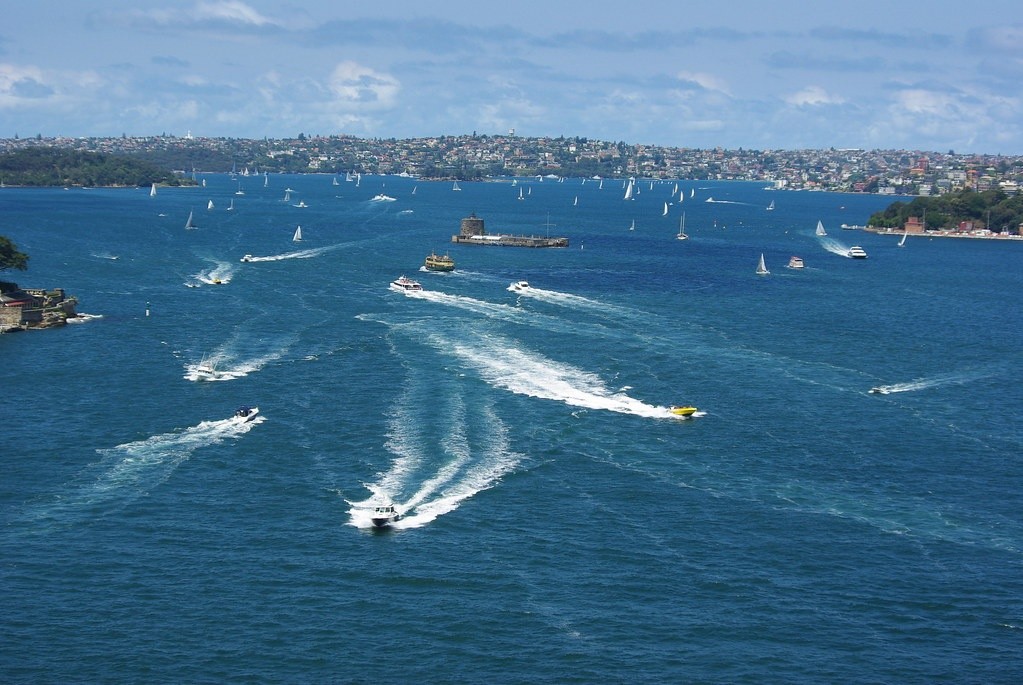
[232,404,259,424]
[212,277,221,284]
[369,504,400,526]
[848,247,867,258]
[389,274,423,293]
[872,386,890,395]
[195,361,215,381]
[425,250,455,271]
[451,213,569,247]
[669,405,698,418]
[515,281,530,292]
[790,257,804,268]
[240,254,258,262]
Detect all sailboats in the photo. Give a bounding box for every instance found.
[754,253,772,275]
[149,160,909,247]
